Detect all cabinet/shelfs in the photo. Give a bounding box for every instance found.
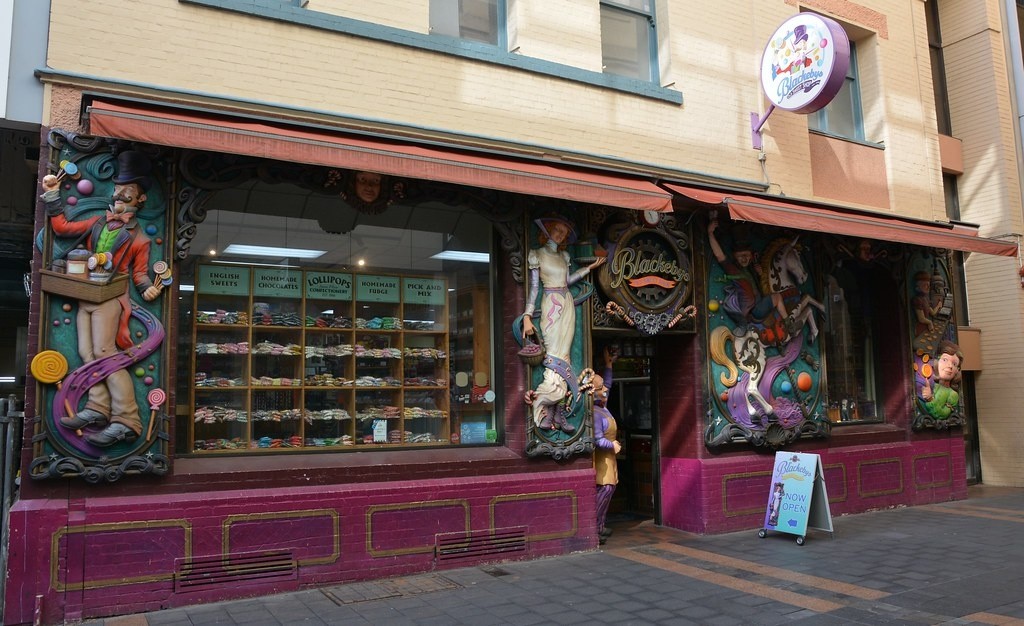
[188,259,451,448]
[606,375,651,438]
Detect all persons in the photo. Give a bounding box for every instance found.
[708,220,788,320]
[860,241,871,260]
[914,272,943,335]
[922,341,964,420]
[355,172,381,202]
[523,221,605,430]
[592,348,622,543]
[43,151,162,447]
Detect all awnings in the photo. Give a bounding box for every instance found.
[89,100,673,213]
[663,182,1018,257]
